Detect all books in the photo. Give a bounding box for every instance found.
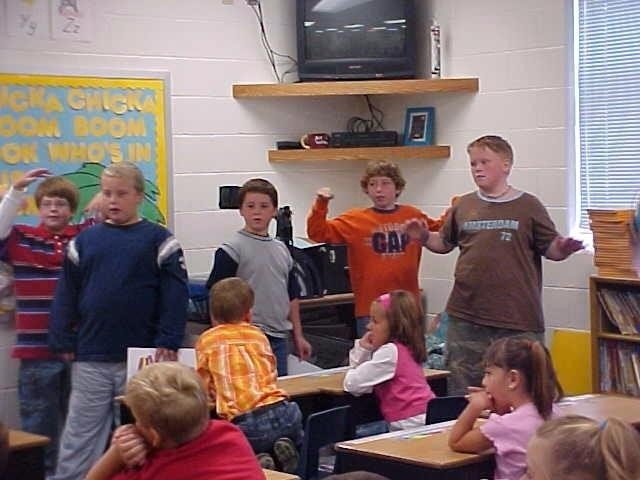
[587,206,639,400]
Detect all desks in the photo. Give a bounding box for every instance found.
[4,427,51,478]
[334,394,640,477]
[115,363,452,433]
[262,467,302,480]
[297,291,426,309]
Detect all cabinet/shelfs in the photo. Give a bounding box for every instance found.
[588,274,640,398]
[232,76,480,158]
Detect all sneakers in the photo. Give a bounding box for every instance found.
[256,452,275,470]
[274,438,300,474]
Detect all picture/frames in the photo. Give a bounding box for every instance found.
[400,106,436,147]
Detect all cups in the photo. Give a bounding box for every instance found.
[299,132,330,150]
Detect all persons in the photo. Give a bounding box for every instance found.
[194,275,305,475]
[46,159,192,480]
[203,177,312,375]
[399,134,587,396]
[518,415,640,480]
[85,361,268,480]
[343,288,436,431]
[0,167,102,480]
[306,159,461,338]
[448,334,566,480]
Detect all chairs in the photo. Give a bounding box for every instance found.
[424,395,468,424]
[294,404,357,479]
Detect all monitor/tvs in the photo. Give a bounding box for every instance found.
[296,0,417,81]
